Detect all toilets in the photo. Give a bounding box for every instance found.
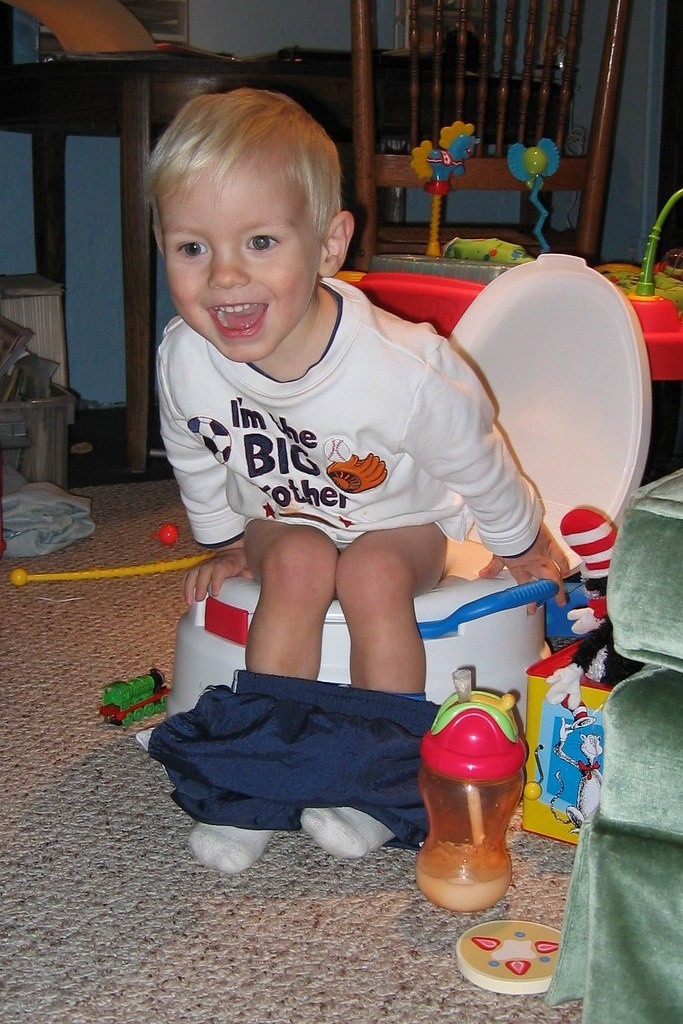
[133,251,655,758]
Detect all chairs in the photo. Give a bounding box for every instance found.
[348,0,628,270]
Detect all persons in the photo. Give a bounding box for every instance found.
[144,90,569,875]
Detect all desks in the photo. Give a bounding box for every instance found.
[0,47,578,474]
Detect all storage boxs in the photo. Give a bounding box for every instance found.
[0,271,83,493]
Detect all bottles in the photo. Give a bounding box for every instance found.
[414,668,530,913]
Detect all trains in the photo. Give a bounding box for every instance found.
[99,667,172,727]
